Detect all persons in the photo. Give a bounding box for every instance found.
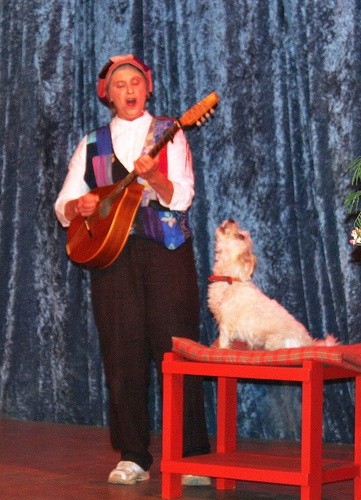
[55,54,213,485]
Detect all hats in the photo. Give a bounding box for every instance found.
[97,54,154,106]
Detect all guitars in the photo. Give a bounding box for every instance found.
[67,93,220,270]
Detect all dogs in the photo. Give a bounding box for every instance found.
[207,218,345,350]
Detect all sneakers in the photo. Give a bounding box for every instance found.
[182,472,211,487]
[107,460,150,485]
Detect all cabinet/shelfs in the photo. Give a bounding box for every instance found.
[159,351,361,500]
